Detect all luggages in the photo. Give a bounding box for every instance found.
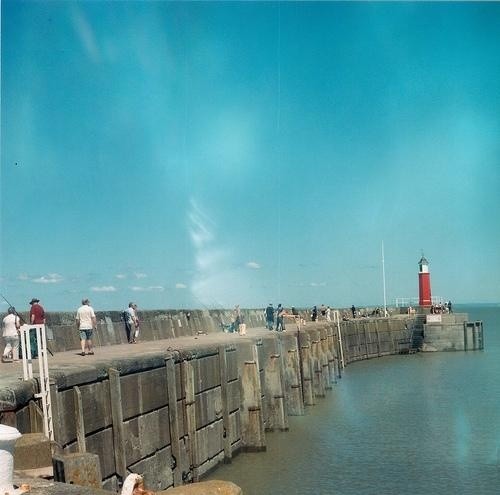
[239,323,246,336]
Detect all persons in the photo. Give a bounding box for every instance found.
[123,302,137,344]
[29,298,46,358]
[74,298,98,357]
[228,300,455,337]
[132,305,140,342]
[1,306,21,363]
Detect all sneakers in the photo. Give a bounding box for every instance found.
[82,352,94,356]
[2,354,19,362]
[128,339,136,344]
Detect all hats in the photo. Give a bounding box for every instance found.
[8,306,15,313]
[29,298,40,304]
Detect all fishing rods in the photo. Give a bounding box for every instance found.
[192,293,232,330]
[0,293,55,357]
